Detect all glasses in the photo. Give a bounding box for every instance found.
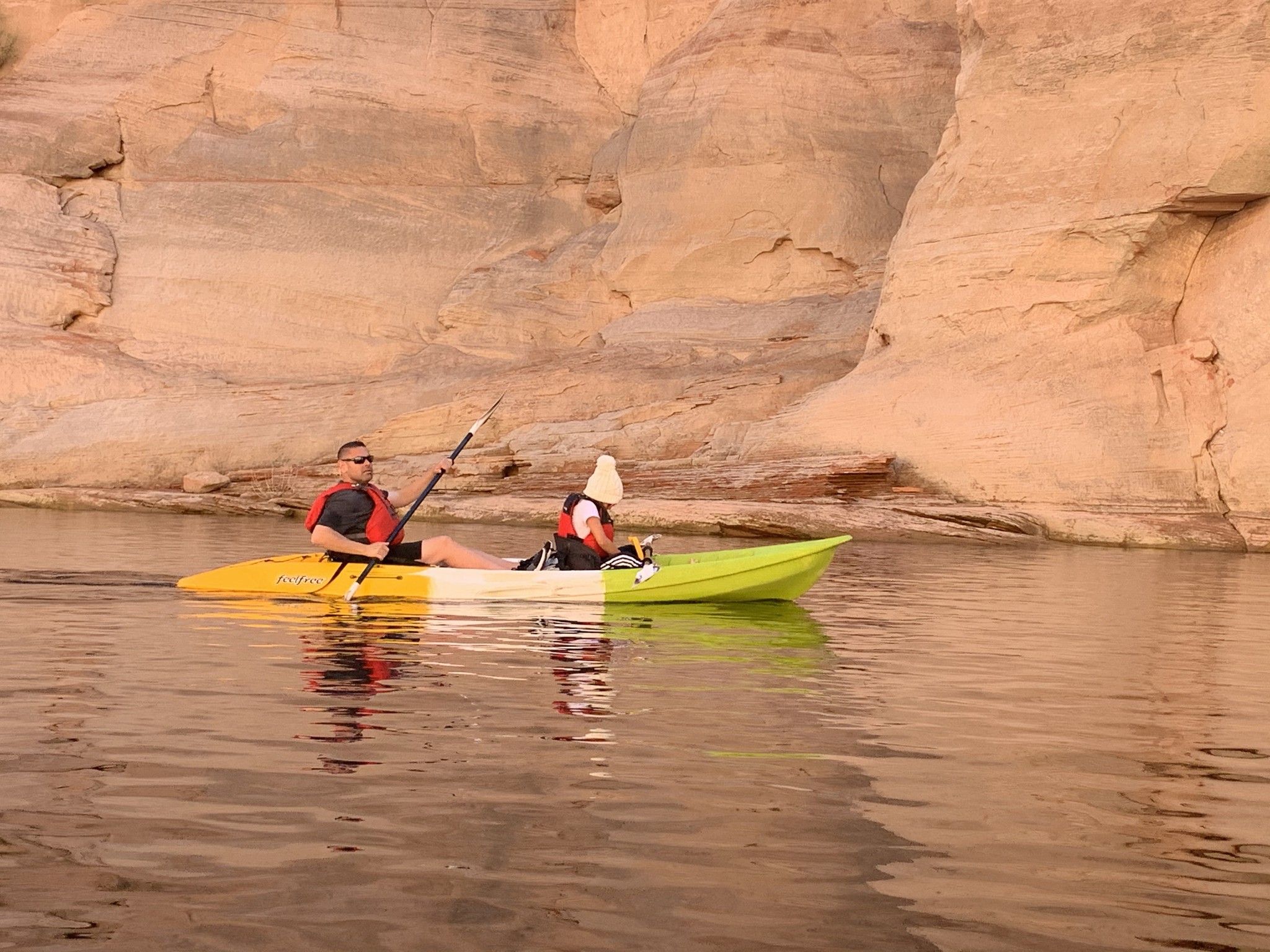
[340,453,375,464]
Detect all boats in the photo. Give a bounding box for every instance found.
[174,533,855,606]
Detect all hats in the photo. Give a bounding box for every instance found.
[582,454,624,506]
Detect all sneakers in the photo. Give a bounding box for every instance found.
[516,540,553,570]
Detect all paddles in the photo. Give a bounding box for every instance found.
[345,388,509,602]
[630,534,662,590]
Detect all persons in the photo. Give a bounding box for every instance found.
[310,441,553,572]
[558,455,643,569]
